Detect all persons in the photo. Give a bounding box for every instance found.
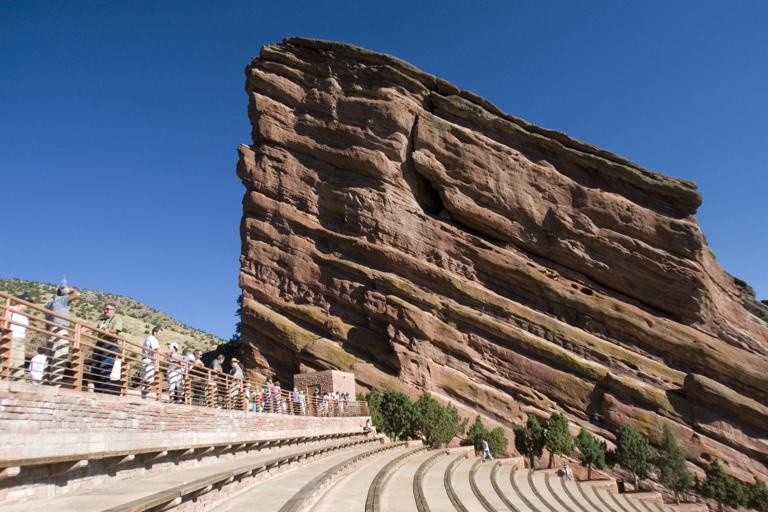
[28,346,49,386]
[563,463,571,481]
[224,357,245,409]
[70,302,123,398]
[242,375,352,418]
[365,418,376,433]
[139,326,164,401]
[481,439,494,462]
[4,293,37,384]
[44,285,81,387]
[165,343,185,404]
[200,353,226,408]
[174,349,205,403]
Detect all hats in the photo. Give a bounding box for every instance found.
[167,342,179,351]
[315,388,319,393]
[231,358,240,362]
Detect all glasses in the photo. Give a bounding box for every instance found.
[105,308,113,311]
